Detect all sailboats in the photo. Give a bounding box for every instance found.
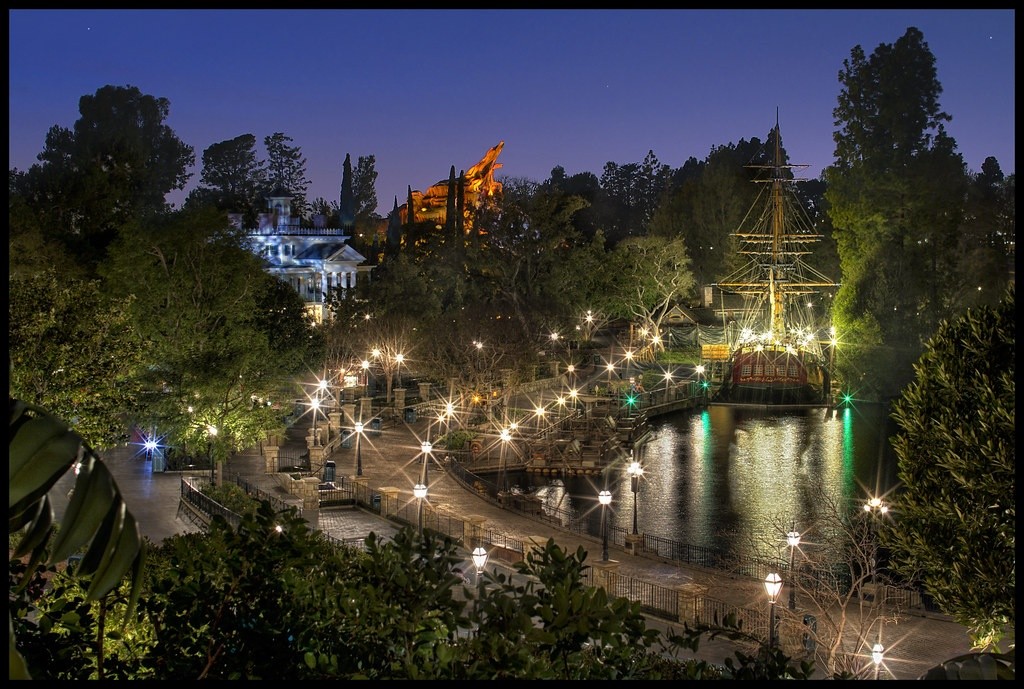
[703,102,849,418]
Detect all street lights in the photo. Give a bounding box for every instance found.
[318,379,328,420]
[311,398,323,445]
[354,417,364,476]
[395,353,404,389]
[471,539,490,601]
[871,634,886,680]
[208,426,219,487]
[420,435,432,486]
[762,562,785,653]
[413,474,428,545]
[498,428,513,493]
[863,496,890,583]
[362,360,370,398]
[626,462,644,535]
[596,487,613,562]
[785,520,802,609]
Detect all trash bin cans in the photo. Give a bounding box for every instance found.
[370,494,381,514]
[296,399,305,414]
[284,411,295,428]
[803,615,817,647]
[405,407,417,423]
[324,460,336,481]
[341,430,353,447]
[594,352,600,364]
[371,417,384,436]
[151,445,165,473]
